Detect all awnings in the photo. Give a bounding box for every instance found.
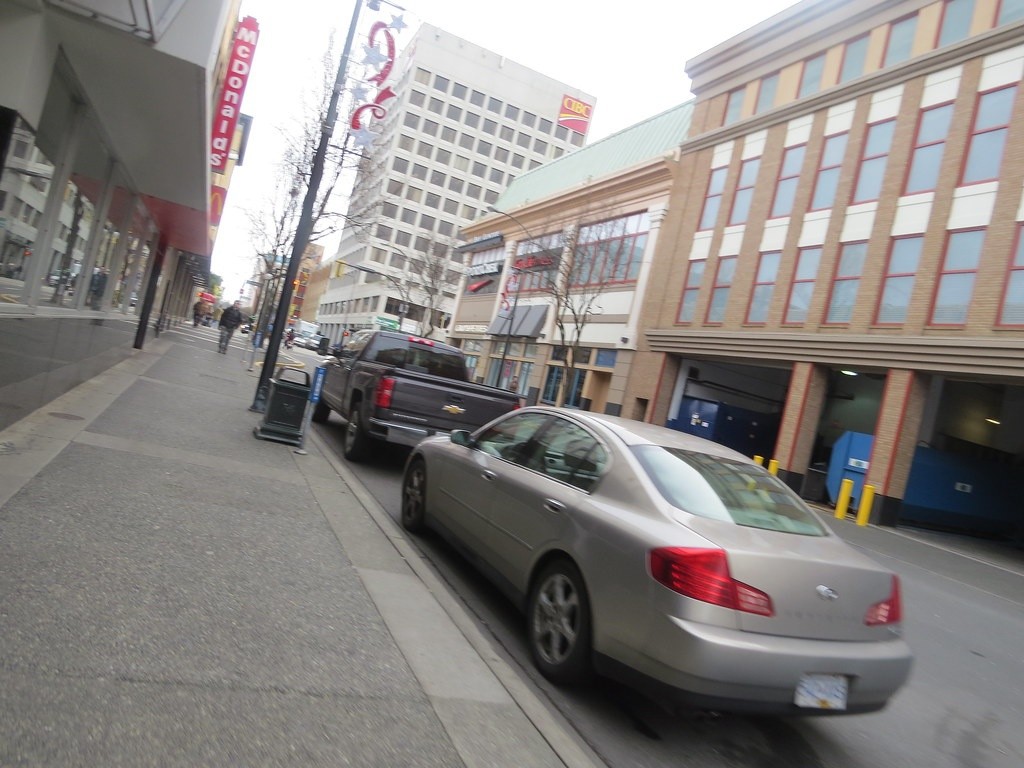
[485,304,549,339]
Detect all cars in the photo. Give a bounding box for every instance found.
[280,319,323,353]
[397,404,915,721]
[47,270,74,291]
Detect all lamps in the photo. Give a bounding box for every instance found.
[985,417,1002,425]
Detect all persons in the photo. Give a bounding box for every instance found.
[192,298,206,327]
[508,376,519,392]
[218,300,243,354]
[285,329,296,347]
[89,266,107,311]
[71,273,79,288]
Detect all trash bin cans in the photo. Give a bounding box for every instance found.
[254,364,312,446]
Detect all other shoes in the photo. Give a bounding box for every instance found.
[219,348,226,354]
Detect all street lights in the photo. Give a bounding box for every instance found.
[250,0,384,414]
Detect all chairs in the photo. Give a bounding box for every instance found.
[554,440,597,487]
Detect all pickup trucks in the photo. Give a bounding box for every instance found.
[307,328,527,466]
